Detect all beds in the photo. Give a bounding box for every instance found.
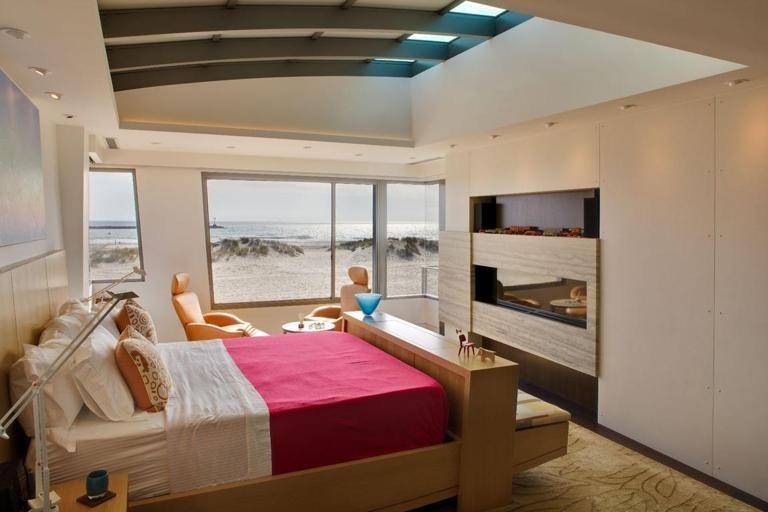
[22,331,462,512]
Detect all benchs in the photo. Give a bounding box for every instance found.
[512,389,571,477]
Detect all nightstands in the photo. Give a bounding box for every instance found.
[49,471,129,512]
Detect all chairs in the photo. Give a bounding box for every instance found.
[171,272,271,341]
[496,280,541,308]
[304,267,372,331]
[566,286,586,317]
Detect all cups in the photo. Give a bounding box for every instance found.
[353,292,383,316]
[86,470,110,502]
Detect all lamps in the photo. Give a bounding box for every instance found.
[0,290,138,512]
[81,266,146,302]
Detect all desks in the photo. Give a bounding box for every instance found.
[282,321,335,334]
[550,299,586,314]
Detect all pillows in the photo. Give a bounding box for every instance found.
[10,297,172,454]
